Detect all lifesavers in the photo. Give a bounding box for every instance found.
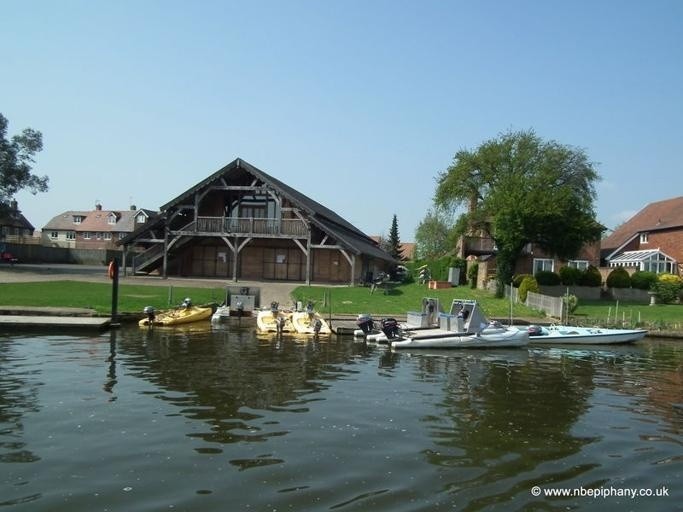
[109,262,114,278]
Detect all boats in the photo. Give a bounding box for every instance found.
[288,300,332,335]
[137,297,213,327]
[257,301,297,333]
[352,297,648,349]
